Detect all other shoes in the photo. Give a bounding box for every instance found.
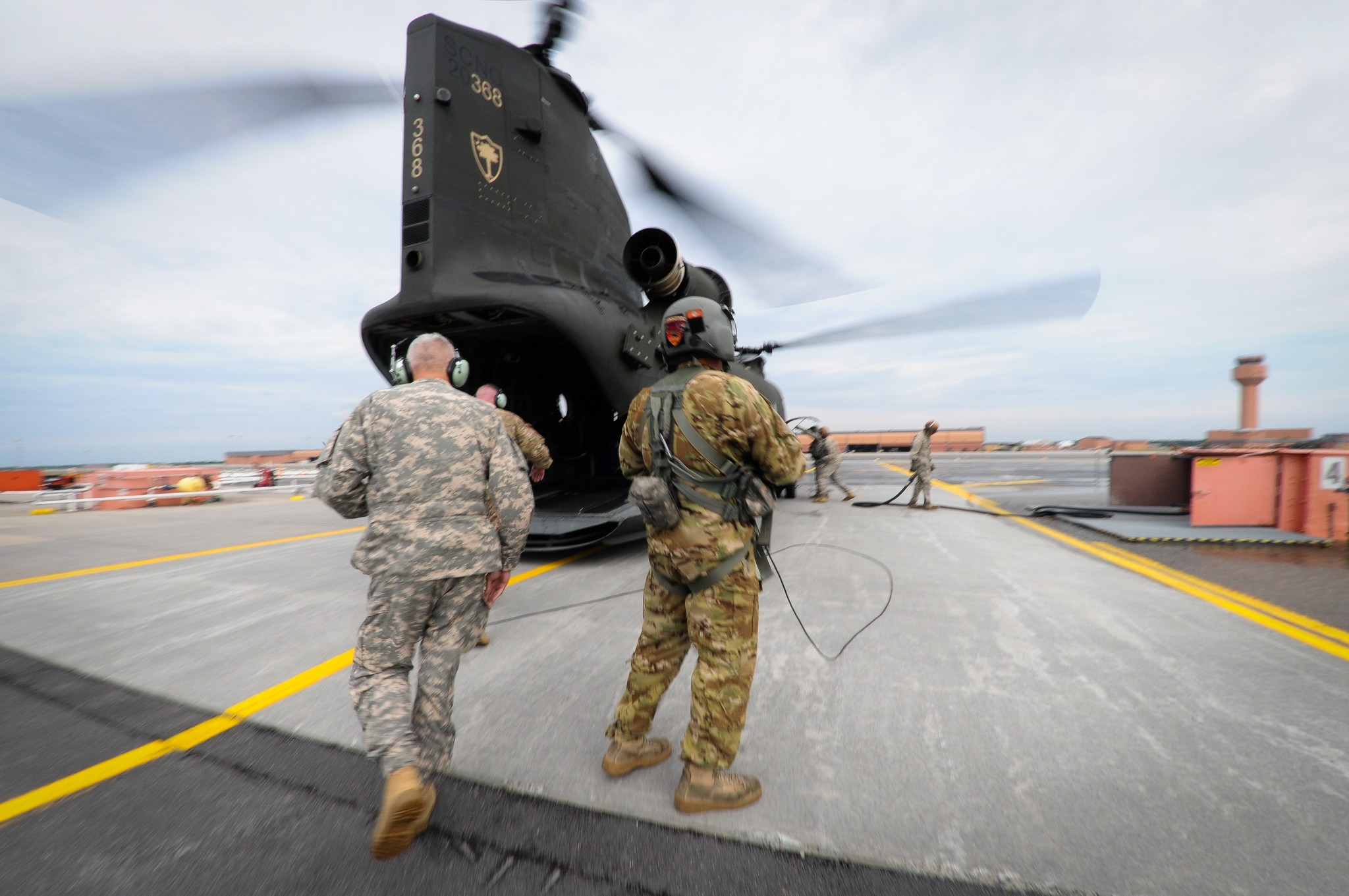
[417,782,437,830]
[814,495,828,502]
[927,505,938,509]
[843,491,855,501]
[477,634,490,645]
[909,503,918,508]
[372,767,424,857]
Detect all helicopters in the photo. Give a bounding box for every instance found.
[0,1,1105,555]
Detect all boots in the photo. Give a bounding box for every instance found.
[601,734,671,777]
[674,759,762,813]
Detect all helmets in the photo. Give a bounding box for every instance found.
[660,298,733,371]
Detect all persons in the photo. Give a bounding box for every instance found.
[474,386,552,647]
[808,426,822,498]
[602,297,805,813]
[909,421,939,509]
[814,427,855,502]
[312,332,535,864]
